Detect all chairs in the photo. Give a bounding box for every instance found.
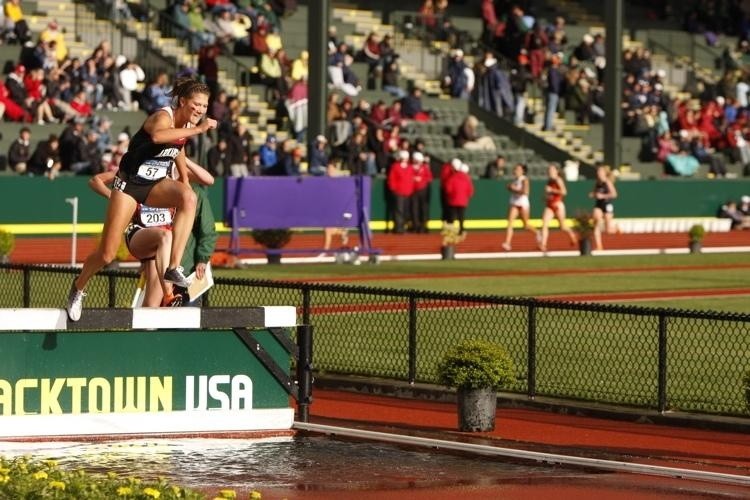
[134,0,750,175]
[0,24,134,176]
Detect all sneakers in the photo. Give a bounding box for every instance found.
[66,276,84,322]
[164,265,191,288]
[165,295,183,307]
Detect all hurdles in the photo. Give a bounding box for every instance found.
[0,305,297,448]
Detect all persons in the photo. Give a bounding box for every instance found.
[67,71,218,321]
[88,155,214,307]
[308,1,749,257]
[1,1,308,176]
[159,181,217,307]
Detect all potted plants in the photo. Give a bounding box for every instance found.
[437,219,461,260]
[435,337,516,433]
[253,226,292,263]
[569,208,599,256]
[687,221,708,256]
